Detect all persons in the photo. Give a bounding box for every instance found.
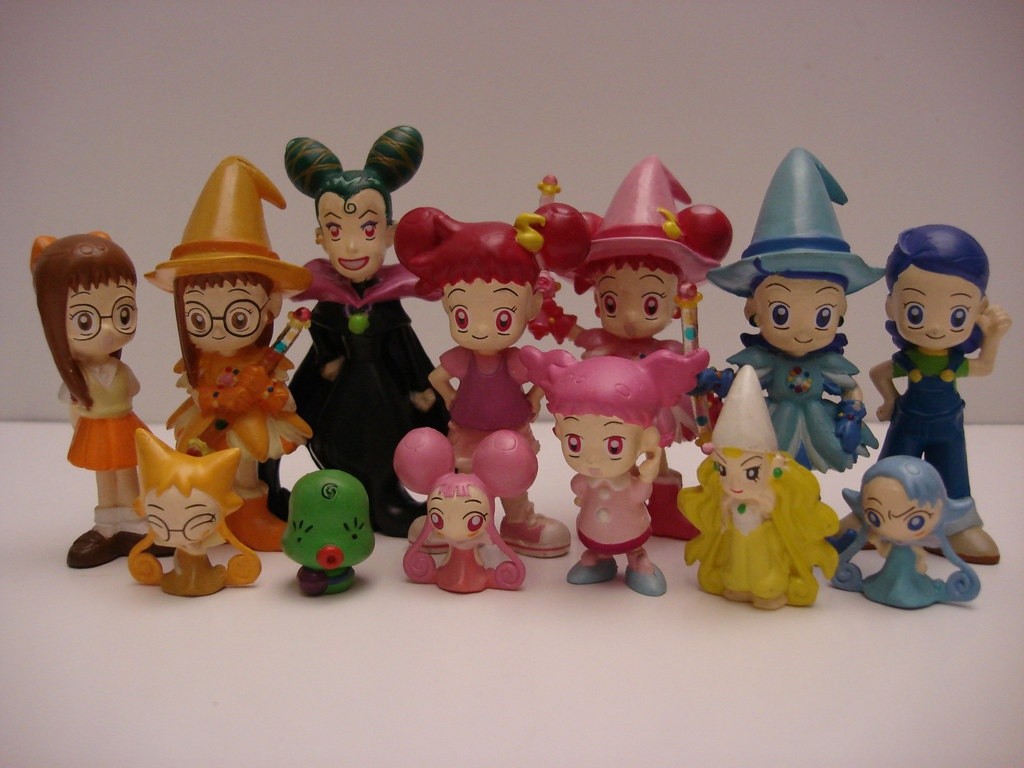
[27,229,175,568]
[255,126,457,540]
[519,343,711,597]
[142,152,312,522]
[391,425,537,595]
[686,145,886,474]
[835,225,1011,565]
[390,204,591,559]
[526,153,733,541]
[123,428,262,598]
[829,452,982,610]
[678,364,841,610]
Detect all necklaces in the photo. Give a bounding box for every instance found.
[345,304,374,333]
